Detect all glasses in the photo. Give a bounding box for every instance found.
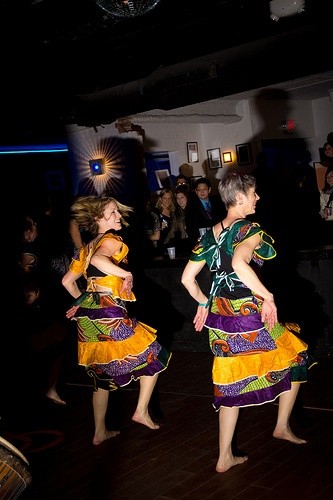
[196,188,208,192]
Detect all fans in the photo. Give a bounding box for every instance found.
[96,0,161,17]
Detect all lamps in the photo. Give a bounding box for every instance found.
[223,152,232,163]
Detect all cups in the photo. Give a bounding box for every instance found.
[167,247,176,259]
[199,227,210,237]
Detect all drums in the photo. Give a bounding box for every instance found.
[0,437,32,500]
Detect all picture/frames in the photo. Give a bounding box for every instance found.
[187,142,199,163]
[190,176,202,183]
[236,143,253,166]
[154,169,170,189]
[207,147,222,170]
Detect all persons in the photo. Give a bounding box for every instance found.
[0,134,333,406]
[62,195,172,445]
[180,170,308,473]
